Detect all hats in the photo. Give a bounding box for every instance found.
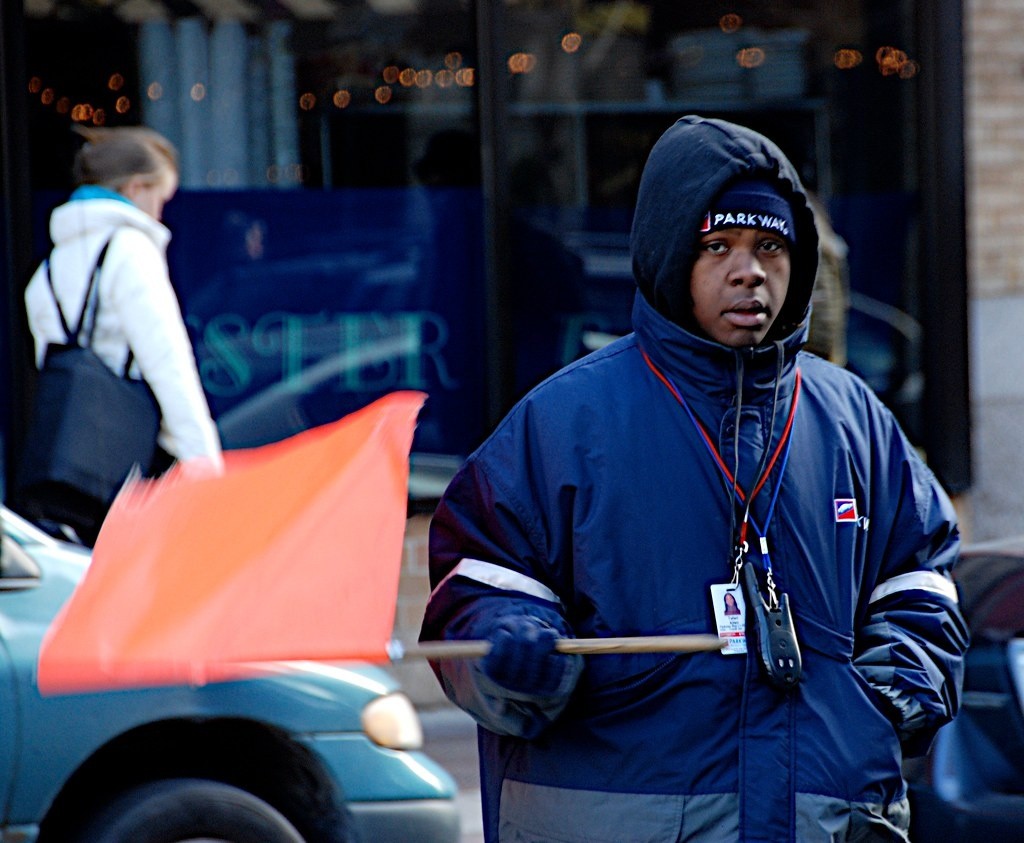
[697,173,796,242]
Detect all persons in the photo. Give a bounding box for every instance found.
[24,130,225,548]
[724,593,741,616]
[416,113,966,843]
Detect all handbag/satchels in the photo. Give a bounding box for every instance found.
[24,241,162,509]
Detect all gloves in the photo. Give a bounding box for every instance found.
[487,613,568,701]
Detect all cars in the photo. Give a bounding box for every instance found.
[0,500,467,841]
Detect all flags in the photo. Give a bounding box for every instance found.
[36,389,425,693]
